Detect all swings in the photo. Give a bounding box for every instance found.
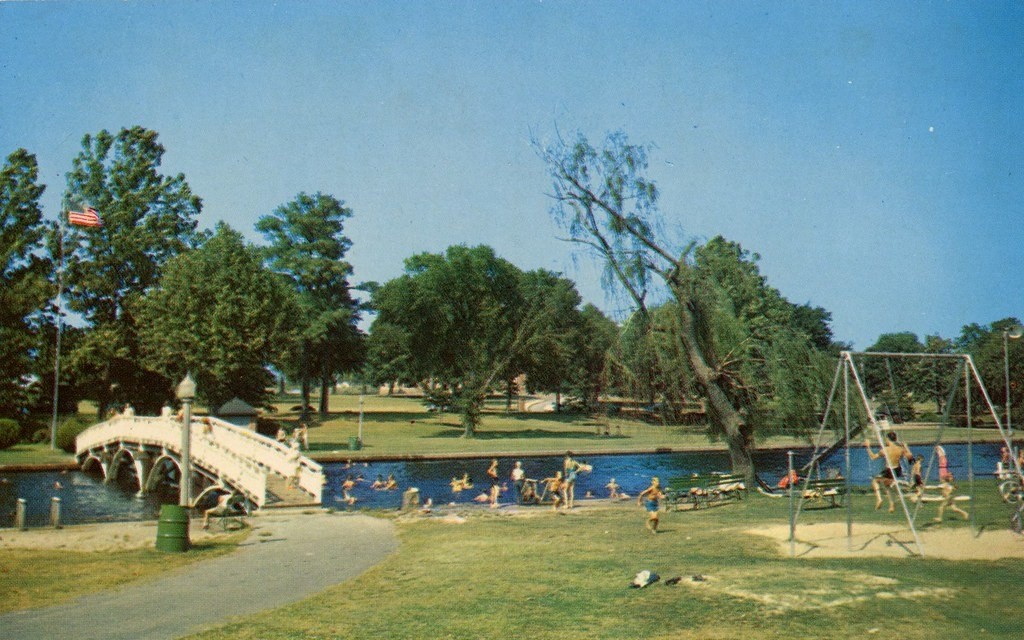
[860,355,915,492]
[916,358,964,490]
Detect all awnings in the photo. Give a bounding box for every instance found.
[487,459,501,509]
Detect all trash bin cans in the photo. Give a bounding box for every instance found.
[348,437,358,451]
[156,503,189,553]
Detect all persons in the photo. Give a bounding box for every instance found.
[910,454,926,504]
[638,477,667,534]
[356,474,365,482]
[511,461,525,504]
[474,489,488,503]
[499,482,509,492]
[342,474,356,487]
[925,474,969,523]
[864,432,914,513]
[449,473,475,492]
[370,474,383,488]
[605,478,620,488]
[934,445,954,483]
[124,403,135,416]
[385,473,399,490]
[560,451,582,509]
[161,400,171,417]
[543,471,563,511]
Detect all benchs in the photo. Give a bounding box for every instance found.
[796,478,847,512]
[662,473,748,511]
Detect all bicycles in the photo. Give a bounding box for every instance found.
[999,469,1024,504]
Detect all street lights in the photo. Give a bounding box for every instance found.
[1002,323,1024,438]
[175,372,196,505]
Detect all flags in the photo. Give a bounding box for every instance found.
[66,204,104,227]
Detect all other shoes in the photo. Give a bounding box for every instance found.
[628,570,660,589]
[692,574,705,581]
[665,576,680,585]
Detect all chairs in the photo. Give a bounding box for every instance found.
[209,489,250,535]
